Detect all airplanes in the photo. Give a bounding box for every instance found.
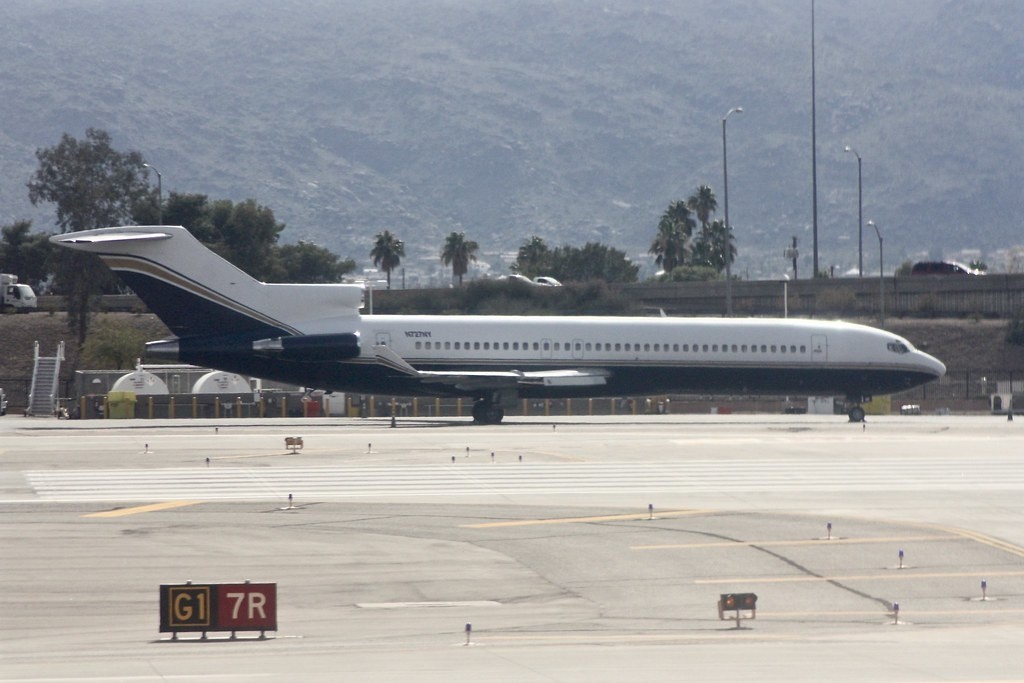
[49,225,946,426]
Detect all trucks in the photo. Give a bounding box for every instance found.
[0,273,38,315]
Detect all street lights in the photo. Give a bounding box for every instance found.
[142,163,164,225]
[844,145,863,278]
[723,106,744,316]
[868,220,885,330]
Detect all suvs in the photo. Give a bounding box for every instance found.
[911,258,986,277]
